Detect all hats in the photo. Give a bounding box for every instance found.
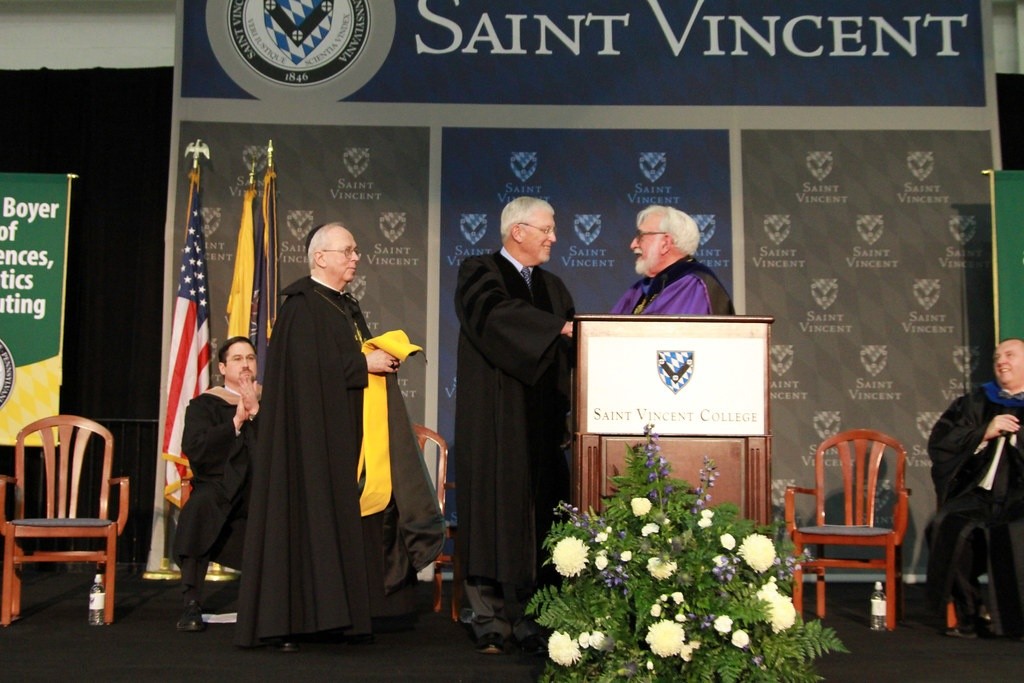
[305,223,327,256]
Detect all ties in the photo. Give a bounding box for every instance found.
[521,266,531,289]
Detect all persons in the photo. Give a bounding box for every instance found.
[613,204,734,316]
[171,336,264,632]
[918,337,1024,640]
[454,196,580,657]
[235,224,447,652]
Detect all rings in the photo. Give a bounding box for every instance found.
[391,361,394,367]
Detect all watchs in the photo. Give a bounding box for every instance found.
[248,413,256,421]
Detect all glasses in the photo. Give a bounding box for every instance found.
[518,222,557,234]
[636,230,674,244]
[320,248,361,258]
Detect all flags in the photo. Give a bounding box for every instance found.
[226,174,280,362]
[162,183,212,507]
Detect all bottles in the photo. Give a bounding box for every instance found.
[88,573,105,626]
[870,581,887,631]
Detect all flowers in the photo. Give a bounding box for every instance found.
[523,426,851,683]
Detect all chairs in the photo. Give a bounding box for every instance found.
[411,426,470,619]
[0,413,131,626]
[785,427,909,628]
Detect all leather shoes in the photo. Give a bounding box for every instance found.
[264,635,296,650]
[177,596,205,632]
[523,636,550,655]
[475,632,504,654]
[297,630,374,646]
